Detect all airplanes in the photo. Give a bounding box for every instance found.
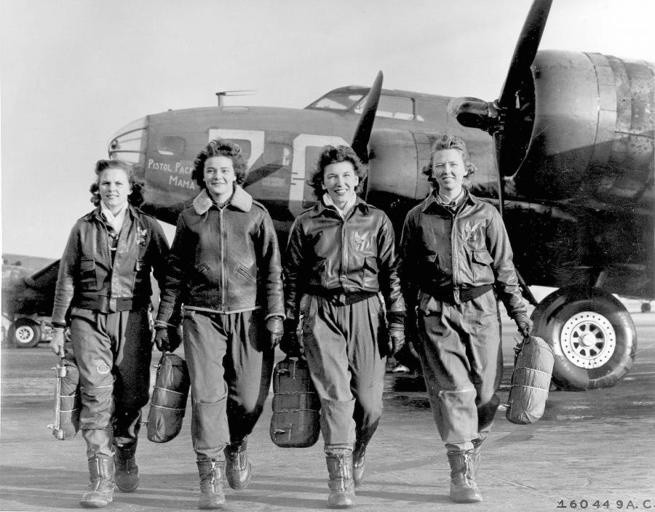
[1,1,655,390]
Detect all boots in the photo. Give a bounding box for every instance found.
[324,447,356,509]
[223,436,252,491]
[354,440,367,483]
[80,455,115,508]
[196,458,226,510]
[114,438,139,492]
[447,449,482,503]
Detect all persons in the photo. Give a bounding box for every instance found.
[50,134,534,508]
